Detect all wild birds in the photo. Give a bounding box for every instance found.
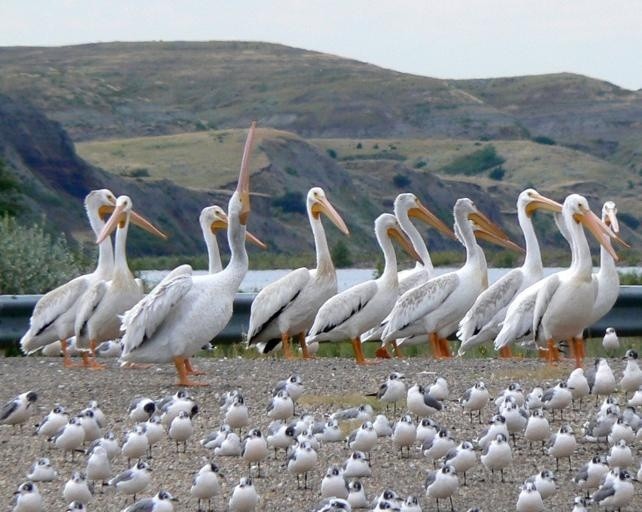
[17,121,632,385]
[1,323,640,512]
